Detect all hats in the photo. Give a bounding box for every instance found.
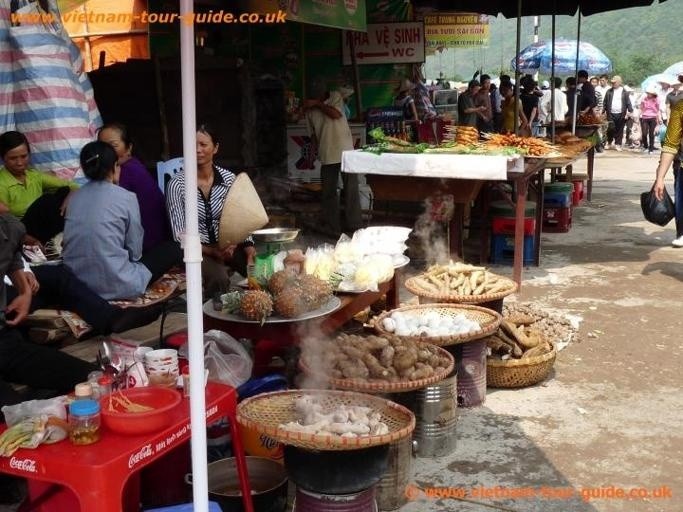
[611,75,622,83]
[541,80,549,89]
[217,171,270,250]
[670,80,681,88]
[658,78,671,88]
[645,87,657,96]
[624,85,634,92]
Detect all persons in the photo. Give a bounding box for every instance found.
[163,121,258,300]
[651,96,683,248]
[59,141,152,303]
[379,66,683,156]
[93,123,172,283]
[2,205,134,406]
[0,131,81,262]
[301,79,362,240]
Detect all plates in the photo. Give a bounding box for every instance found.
[250,227,300,243]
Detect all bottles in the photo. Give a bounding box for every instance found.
[368,120,407,144]
[87,372,111,399]
[66,382,101,446]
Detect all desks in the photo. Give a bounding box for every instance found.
[1,381,254,512]
[545,138,598,202]
[341,145,545,293]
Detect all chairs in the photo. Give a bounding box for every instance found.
[155,156,187,348]
[31,263,185,350]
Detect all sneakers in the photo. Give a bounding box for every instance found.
[671,235,683,249]
[625,139,659,155]
[615,145,623,153]
[604,143,611,150]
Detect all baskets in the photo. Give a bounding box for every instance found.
[484,340,558,389]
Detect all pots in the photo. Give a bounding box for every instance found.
[178,452,289,511]
[537,123,602,139]
[282,441,389,495]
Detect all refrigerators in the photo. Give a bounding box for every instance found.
[285,121,367,184]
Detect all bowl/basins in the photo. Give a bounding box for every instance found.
[144,348,179,386]
[98,386,181,434]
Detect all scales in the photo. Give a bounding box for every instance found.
[249,227,300,281]
